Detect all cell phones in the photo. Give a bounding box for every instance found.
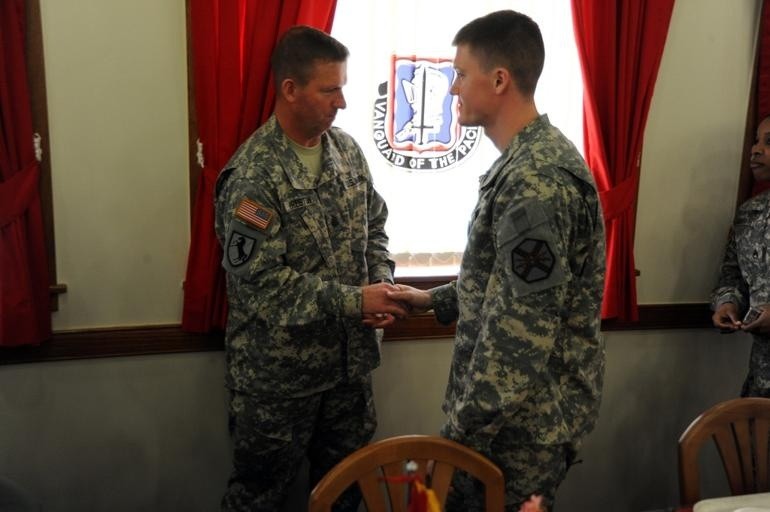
[742,306,762,325]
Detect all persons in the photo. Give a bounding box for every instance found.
[210,24,412,512]
[385,7,612,511]
[708,112,770,401]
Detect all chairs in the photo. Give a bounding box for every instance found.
[677,397,769,512]
[310,433,505,512]
